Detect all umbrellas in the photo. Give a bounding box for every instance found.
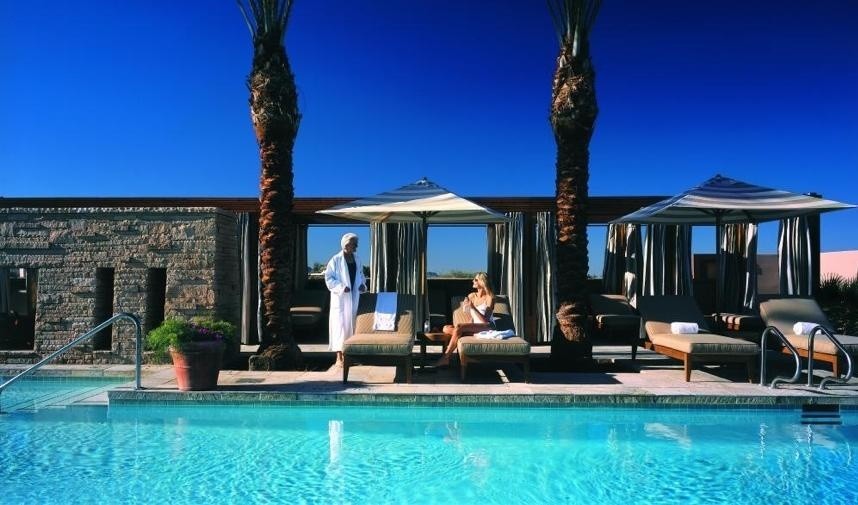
[313,174,514,376]
[605,174,858,340]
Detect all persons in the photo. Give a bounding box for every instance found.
[326,419,346,488]
[325,231,368,370]
[446,419,463,446]
[430,272,495,367]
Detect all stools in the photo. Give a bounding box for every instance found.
[712,313,758,332]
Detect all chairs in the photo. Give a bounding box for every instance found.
[289,288,329,338]
[633,295,759,384]
[450,295,530,383]
[590,295,642,342]
[760,295,857,381]
[343,292,415,383]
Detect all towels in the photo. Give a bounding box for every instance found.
[373,291,399,331]
[474,329,515,340]
[793,322,821,335]
[670,321,699,334]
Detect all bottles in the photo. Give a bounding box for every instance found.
[463,297,470,313]
[423,318,430,332]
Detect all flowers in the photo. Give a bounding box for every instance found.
[151,310,239,366]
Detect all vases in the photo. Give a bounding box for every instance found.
[168,347,226,390]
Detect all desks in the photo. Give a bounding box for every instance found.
[417,332,452,370]
